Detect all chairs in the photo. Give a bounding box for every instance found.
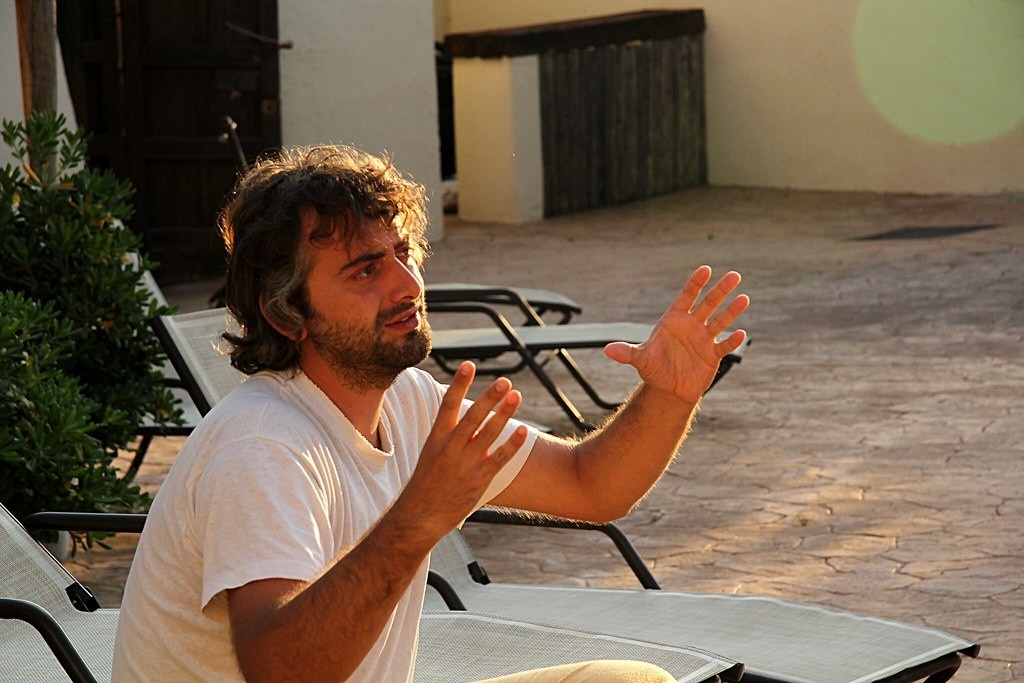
[427,289,752,435]
[0,501,745,683]
[107,214,250,485]
[130,305,979,683]
[424,281,582,376]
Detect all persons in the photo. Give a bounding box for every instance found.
[111,146,751,683]
